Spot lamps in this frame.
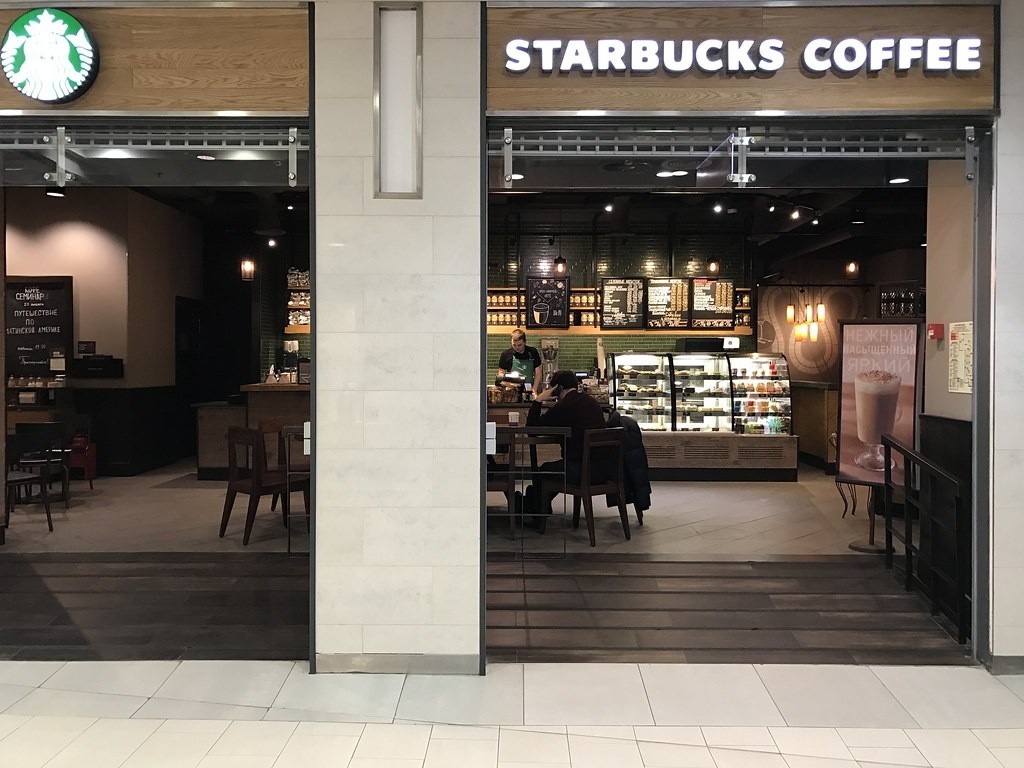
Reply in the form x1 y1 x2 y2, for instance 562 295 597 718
240 249 256 282
785 258 827 342
554 202 566 277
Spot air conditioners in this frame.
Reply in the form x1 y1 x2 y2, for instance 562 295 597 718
841 254 862 287
706 196 720 276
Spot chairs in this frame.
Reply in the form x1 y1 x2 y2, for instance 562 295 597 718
3 432 54 532
483 425 520 542
41 411 96 492
218 424 312 548
602 412 647 526
255 419 311 512
9 421 72 513
536 426 631 548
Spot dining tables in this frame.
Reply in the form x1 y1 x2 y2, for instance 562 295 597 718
487 415 572 535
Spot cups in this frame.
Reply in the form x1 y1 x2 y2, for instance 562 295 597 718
533 303 549 324
508 412 519 426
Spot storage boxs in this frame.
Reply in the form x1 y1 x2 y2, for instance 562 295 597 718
604 351 799 482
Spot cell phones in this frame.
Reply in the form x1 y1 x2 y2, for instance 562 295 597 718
551 385 563 402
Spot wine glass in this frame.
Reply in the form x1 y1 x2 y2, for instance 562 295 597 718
853 374 903 472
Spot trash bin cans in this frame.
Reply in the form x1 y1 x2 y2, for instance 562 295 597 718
68 433 96 480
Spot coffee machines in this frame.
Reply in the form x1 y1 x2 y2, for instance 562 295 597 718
540 337 559 389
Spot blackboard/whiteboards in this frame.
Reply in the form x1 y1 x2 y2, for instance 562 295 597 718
525 275 570 329
4 275 76 380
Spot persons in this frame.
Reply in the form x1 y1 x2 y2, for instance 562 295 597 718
525 369 608 535
497 328 543 401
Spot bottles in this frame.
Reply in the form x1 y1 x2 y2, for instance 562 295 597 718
36 377 43 386
8 376 16 386
18 377 26 385
27 377 36 386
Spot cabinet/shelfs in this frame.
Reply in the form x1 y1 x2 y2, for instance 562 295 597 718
282 270 312 336
485 285 758 339
